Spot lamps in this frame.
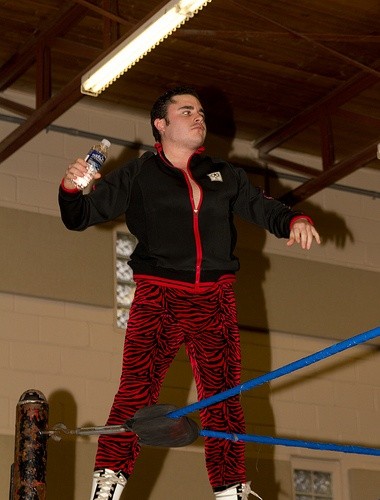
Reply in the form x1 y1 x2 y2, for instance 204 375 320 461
80 0 212 97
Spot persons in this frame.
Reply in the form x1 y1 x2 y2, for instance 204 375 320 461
56 86 321 500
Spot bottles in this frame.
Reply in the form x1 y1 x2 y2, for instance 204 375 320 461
70 139 111 189
